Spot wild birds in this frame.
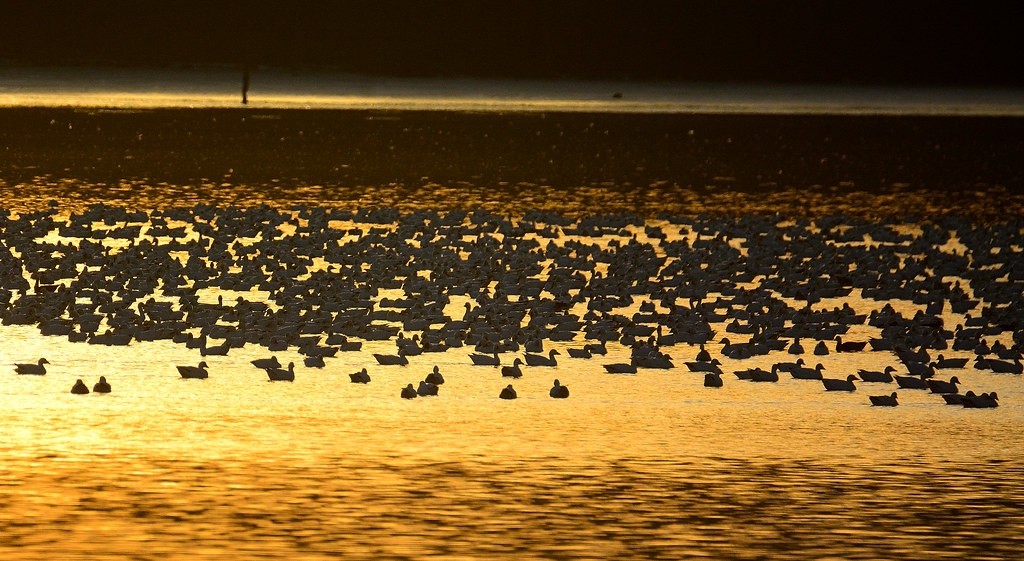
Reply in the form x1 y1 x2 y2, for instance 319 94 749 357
0 171 1024 411
